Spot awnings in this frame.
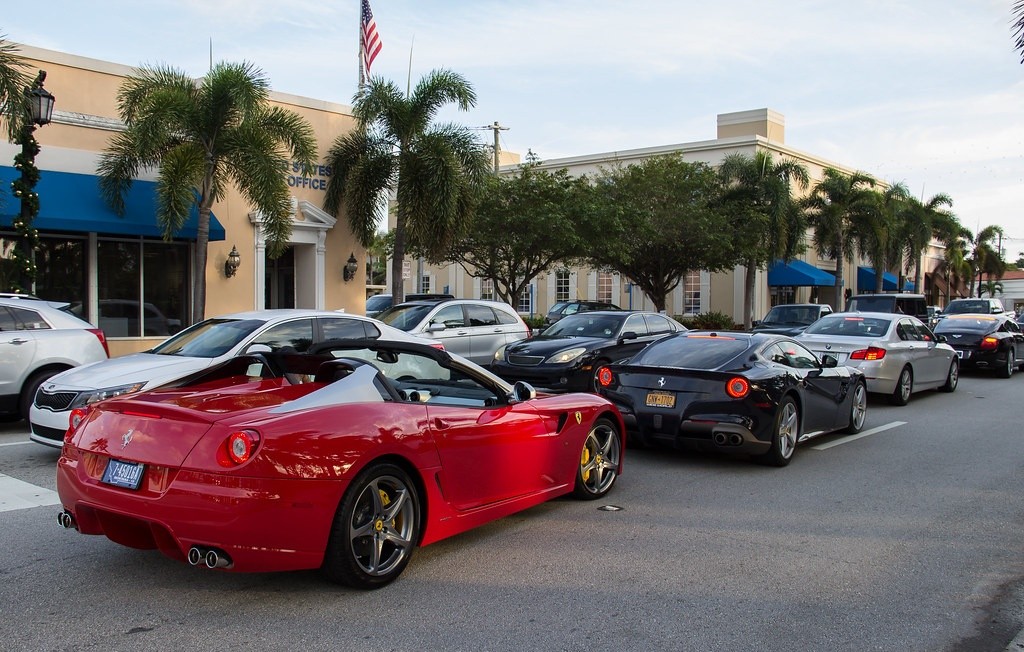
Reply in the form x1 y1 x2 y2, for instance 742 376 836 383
767 257 844 287
950 275 975 297
0 165 226 241
858 266 915 290
926 272 959 297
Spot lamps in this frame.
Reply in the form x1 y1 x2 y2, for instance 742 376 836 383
812 286 818 298
846 288 852 299
225 244 241 278
343 251 358 282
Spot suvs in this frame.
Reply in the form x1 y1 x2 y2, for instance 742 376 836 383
0 293 111 424
537 299 623 335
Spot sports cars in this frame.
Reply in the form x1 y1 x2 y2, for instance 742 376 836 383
598 330 868 468
57 341 628 593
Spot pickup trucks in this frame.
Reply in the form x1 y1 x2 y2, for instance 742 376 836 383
937 299 1016 323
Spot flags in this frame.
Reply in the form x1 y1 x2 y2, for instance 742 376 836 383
362 0 382 72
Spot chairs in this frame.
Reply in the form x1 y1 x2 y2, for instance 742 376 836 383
315 357 402 401
244 351 302 385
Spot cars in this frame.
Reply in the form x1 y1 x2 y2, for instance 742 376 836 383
489 309 688 394
63 299 170 338
367 293 455 325
926 305 942 318
751 305 834 343
791 313 962 406
29 309 447 447
933 314 1024 378
331 298 530 384
1016 313 1024 330
842 293 930 331
